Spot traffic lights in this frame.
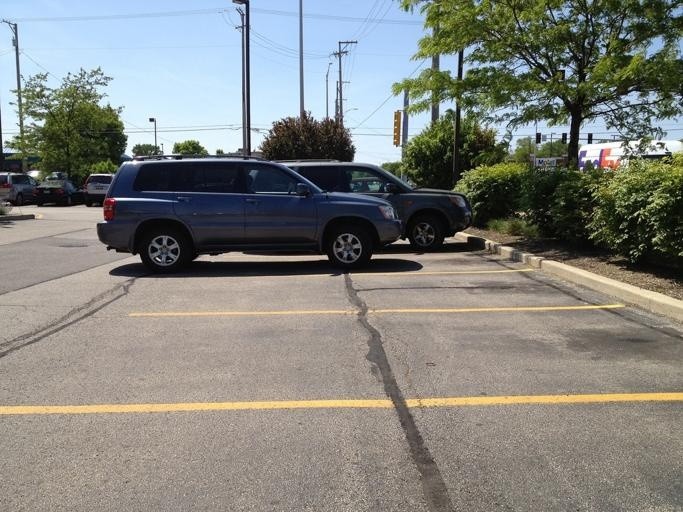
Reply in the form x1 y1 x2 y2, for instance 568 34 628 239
588 133 592 143
536 133 541 144
562 133 567 144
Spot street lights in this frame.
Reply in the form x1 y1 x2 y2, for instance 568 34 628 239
233 0 251 154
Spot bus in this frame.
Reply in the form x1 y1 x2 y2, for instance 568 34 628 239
578 140 674 173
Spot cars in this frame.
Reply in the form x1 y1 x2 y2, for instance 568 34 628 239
0 170 117 215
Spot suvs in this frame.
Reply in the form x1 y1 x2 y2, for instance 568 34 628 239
96 153 405 271
266 158 473 252
